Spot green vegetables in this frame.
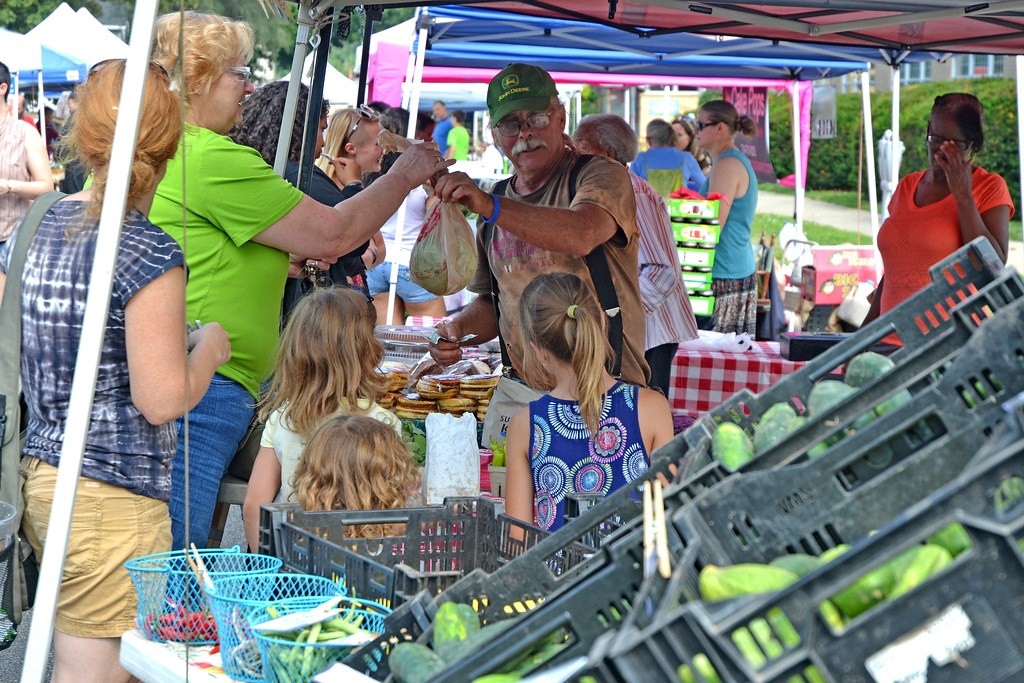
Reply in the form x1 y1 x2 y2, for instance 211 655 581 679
402 421 426 467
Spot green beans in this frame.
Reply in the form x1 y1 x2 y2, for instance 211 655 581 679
261 601 363 683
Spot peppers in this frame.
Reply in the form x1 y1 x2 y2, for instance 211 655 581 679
489 433 507 467
144 606 220 654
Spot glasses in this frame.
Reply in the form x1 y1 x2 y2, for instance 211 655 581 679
346 104 374 142
695 121 726 131
497 109 558 137
926 121 974 150
225 66 251 84
86 58 172 91
317 278 374 304
676 115 694 126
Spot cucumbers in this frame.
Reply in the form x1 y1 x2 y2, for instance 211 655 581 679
388 462 1024 683
712 352 915 471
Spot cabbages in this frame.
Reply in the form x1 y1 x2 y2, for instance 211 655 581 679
410 232 476 295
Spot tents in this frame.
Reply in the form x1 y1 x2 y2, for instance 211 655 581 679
357 16 813 228
18 0 1024 683
351 43 585 135
0 0 132 151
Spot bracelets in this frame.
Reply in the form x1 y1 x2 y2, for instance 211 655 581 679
6 179 16 194
481 193 501 223
347 179 365 189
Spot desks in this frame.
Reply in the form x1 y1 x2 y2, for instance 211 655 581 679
205 477 249 551
403 314 842 431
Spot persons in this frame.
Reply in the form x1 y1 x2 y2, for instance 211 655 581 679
628 117 707 194
367 98 470 325
500 271 680 580
696 100 758 337
855 90 1015 354
79 12 458 602
0 61 91 260
0 59 231 683
225 79 368 330
572 112 699 401
240 288 457 601
316 103 388 295
670 114 712 175
429 60 653 387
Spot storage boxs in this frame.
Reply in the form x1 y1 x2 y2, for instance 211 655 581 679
258 192 1024 683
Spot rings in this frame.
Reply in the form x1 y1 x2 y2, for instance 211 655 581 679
437 156 441 162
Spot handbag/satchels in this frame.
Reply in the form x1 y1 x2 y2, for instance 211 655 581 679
377 131 478 294
0 443 39 651
758 247 785 338
481 375 547 449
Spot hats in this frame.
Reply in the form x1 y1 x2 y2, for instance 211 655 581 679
487 63 559 128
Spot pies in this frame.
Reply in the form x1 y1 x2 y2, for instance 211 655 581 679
382 371 499 420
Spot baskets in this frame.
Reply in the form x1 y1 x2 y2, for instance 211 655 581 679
206 574 346 682
257 233 1024 683
247 596 394 683
125 547 283 643
